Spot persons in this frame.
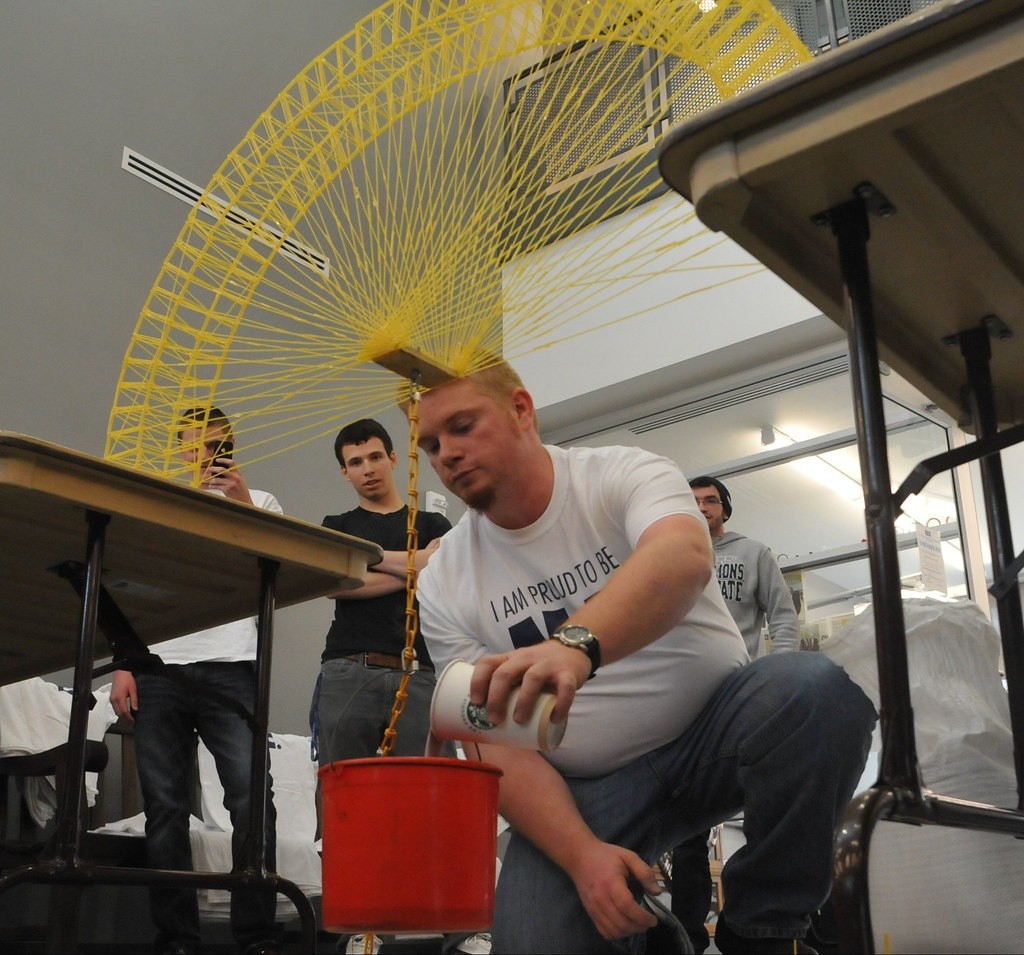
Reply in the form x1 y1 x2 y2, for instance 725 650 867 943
308 419 492 955
107 405 284 954
670 475 802 955
398 346 879 955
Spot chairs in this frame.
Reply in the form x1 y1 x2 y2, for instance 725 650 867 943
61 659 200 954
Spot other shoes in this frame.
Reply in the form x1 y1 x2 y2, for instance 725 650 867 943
247 941 281 955
160 941 194 955
714 914 819 955
642 891 696 955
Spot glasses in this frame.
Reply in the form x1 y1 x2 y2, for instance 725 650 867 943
695 496 724 505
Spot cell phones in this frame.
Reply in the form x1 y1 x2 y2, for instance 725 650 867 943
212 441 233 478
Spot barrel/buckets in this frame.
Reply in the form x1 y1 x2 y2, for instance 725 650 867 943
313 668 506 934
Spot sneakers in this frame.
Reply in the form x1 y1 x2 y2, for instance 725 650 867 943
455 933 492 955
346 934 383 955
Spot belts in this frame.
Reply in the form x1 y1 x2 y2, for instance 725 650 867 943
345 652 434 671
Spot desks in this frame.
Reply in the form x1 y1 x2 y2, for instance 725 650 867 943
651 2 1024 955
1 429 386 955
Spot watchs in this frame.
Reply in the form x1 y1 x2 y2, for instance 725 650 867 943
549 625 601 680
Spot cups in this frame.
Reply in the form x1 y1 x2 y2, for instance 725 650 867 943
430 658 566 752
